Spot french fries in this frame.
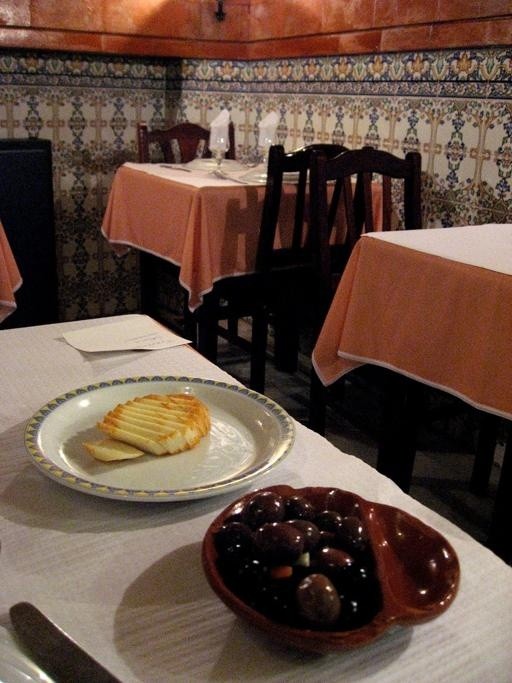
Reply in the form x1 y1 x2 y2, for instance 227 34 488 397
83 390 213 464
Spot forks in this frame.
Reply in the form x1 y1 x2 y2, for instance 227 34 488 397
214 170 247 185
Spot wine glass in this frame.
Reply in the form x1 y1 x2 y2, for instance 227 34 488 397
262 131 281 176
208 132 230 174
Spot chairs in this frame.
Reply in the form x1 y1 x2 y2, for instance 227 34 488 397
308 150 422 438
219 143 376 395
136 121 236 314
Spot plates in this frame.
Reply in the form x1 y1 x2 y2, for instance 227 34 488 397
23 374 297 503
199 483 462 656
284 171 309 181
194 157 233 171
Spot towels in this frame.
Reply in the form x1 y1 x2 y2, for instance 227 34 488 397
208 107 231 152
258 110 280 150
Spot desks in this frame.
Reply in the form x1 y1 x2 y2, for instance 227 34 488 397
1 140 58 325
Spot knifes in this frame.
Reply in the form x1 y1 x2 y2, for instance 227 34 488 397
159 163 192 172
7 600 122 683
